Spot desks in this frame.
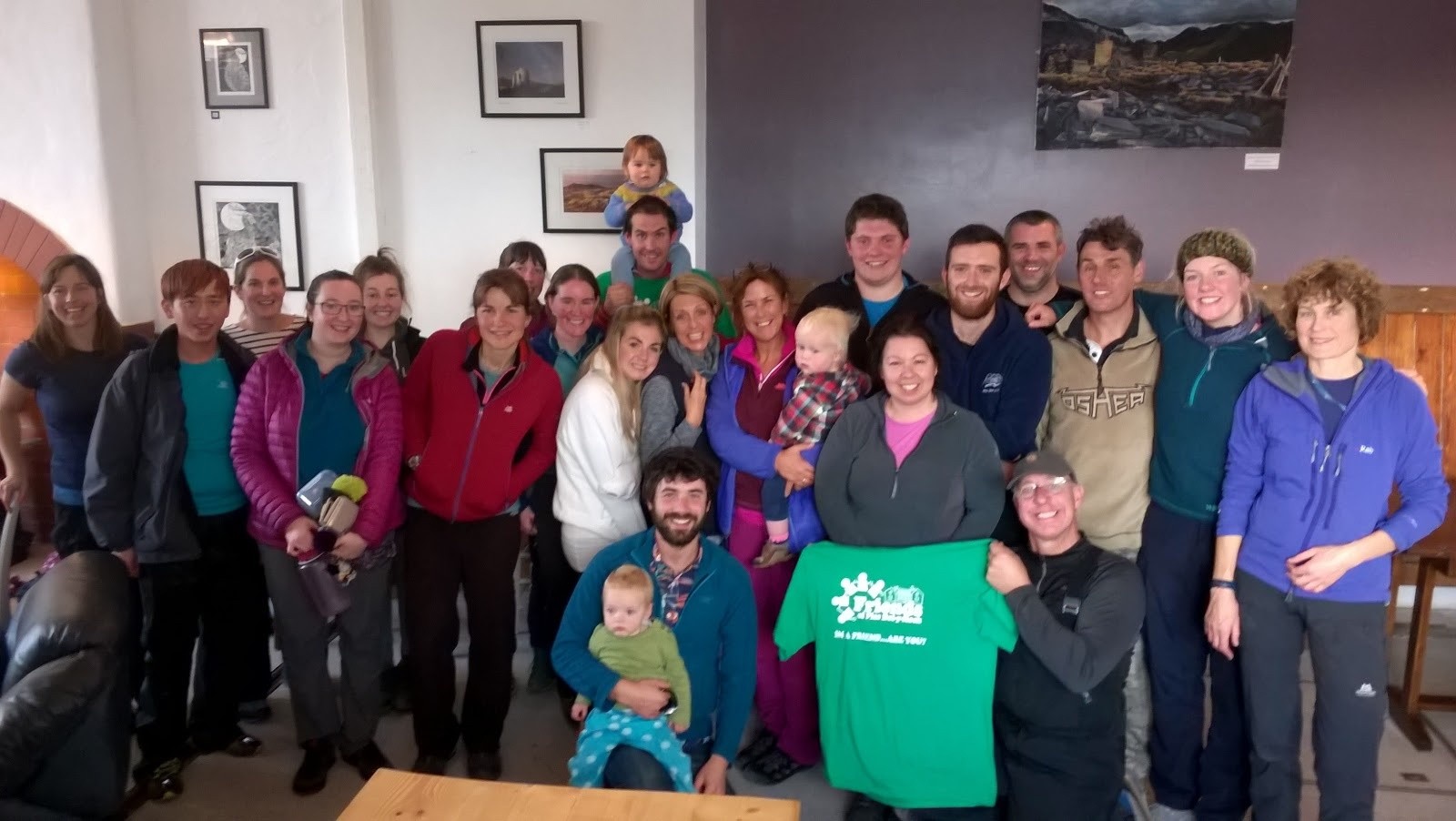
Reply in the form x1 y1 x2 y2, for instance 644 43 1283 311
335 768 801 821
1388 478 1456 753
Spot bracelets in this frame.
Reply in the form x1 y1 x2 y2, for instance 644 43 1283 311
1209 579 1238 590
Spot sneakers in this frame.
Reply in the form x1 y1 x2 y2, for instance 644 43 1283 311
139 749 184 803
192 723 262 757
526 647 557 692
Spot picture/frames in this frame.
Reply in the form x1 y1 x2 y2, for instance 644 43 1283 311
475 19 585 118
195 181 306 291
540 148 635 233
199 28 269 109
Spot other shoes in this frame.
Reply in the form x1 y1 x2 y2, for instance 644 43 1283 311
410 716 460 774
736 733 816 784
461 710 503 780
292 738 336 796
337 723 387 782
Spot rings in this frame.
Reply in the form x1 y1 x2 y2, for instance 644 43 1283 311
803 478 807 484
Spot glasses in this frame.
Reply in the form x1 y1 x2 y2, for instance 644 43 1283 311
234 246 281 271
316 300 366 316
1019 477 1066 497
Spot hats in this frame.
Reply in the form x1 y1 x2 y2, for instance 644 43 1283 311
1006 451 1074 491
1176 227 1256 282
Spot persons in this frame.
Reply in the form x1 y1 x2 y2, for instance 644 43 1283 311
605 135 695 292
0 195 1449 821
